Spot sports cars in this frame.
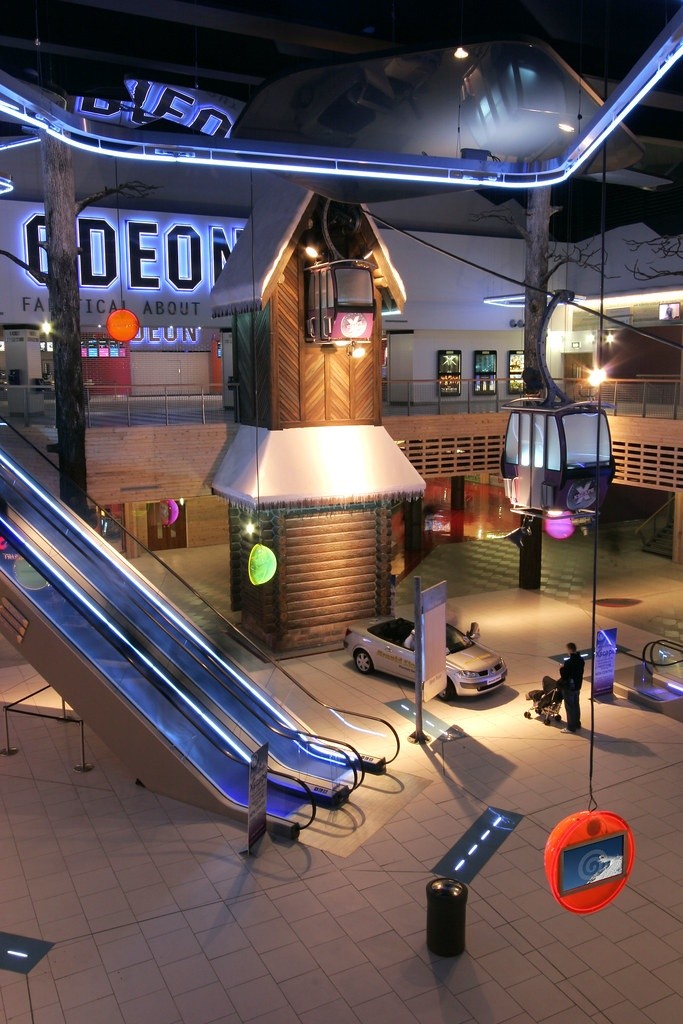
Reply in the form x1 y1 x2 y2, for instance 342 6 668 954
343 614 508 701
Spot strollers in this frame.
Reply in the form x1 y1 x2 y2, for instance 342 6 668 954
524 674 565 725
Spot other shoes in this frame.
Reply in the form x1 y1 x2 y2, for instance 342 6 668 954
561 728 573 734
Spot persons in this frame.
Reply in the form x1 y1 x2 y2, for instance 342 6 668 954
557 642 585 733
